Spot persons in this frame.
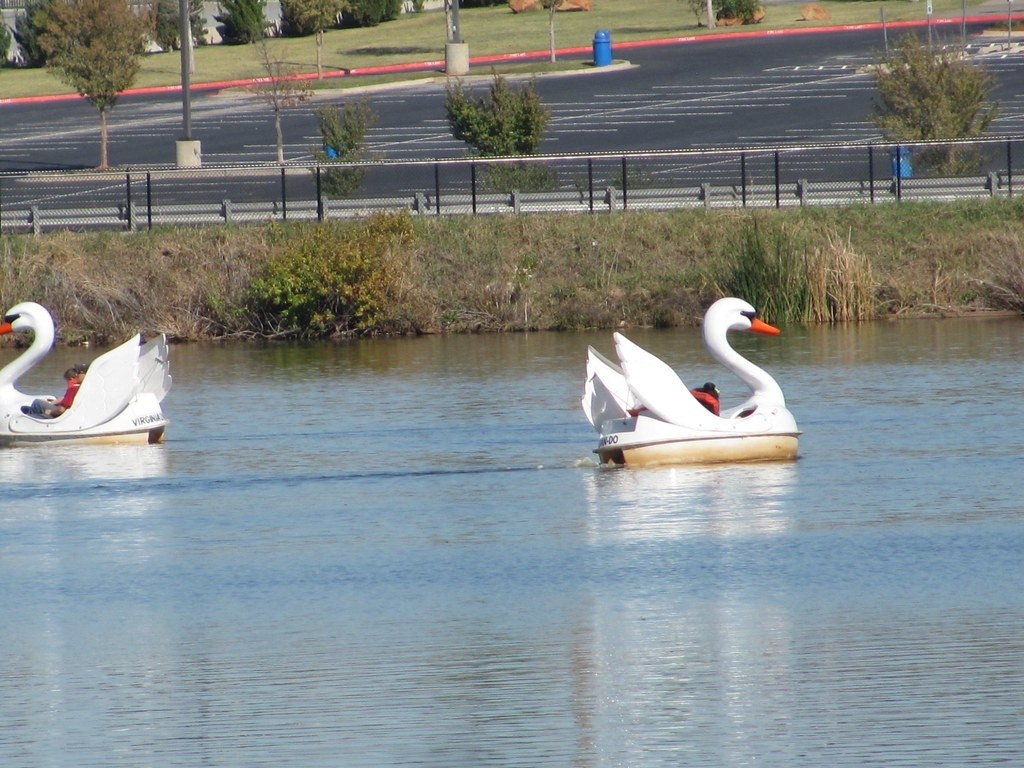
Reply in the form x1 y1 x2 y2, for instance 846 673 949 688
22 364 90 418
41 368 77 404
690 382 720 417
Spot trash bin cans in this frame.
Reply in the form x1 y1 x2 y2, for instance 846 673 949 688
890 147 913 179
593 31 611 67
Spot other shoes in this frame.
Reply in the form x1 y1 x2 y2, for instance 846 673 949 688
21 405 30 412
